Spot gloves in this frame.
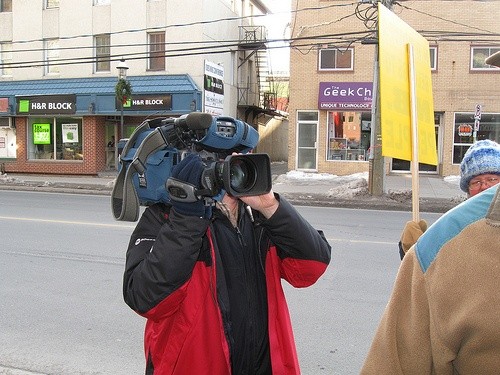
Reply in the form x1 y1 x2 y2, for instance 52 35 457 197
164 153 205 217
400 218 428 254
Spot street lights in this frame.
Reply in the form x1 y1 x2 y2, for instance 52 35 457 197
116 58 129 139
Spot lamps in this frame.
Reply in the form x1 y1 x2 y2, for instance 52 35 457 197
190 100 196 111
88 103 93 113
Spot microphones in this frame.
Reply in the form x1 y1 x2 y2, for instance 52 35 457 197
174 113 212 130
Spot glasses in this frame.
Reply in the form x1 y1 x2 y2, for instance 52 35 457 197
468 177 500 191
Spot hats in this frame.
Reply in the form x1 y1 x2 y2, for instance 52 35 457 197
460 139 500 192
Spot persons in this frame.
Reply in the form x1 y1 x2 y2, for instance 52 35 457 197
122 134 332 375
397 138 500 262
360 181 500 375
